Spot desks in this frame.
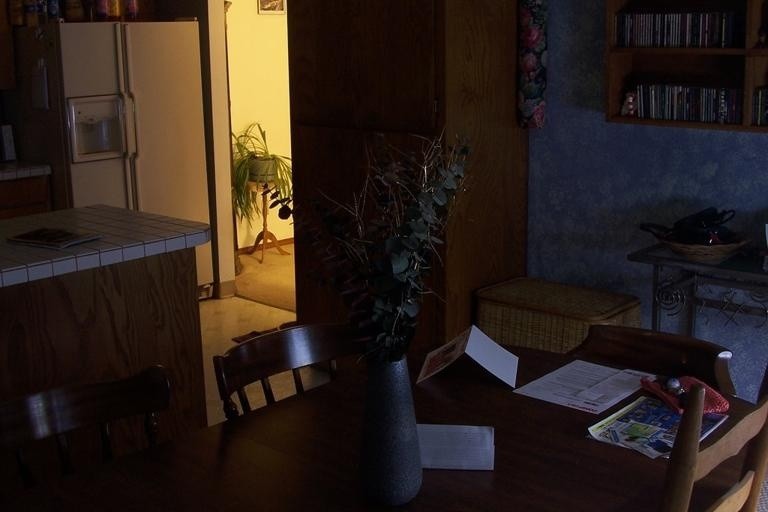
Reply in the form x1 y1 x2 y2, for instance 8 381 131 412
626 239 768 338
3 339 755 507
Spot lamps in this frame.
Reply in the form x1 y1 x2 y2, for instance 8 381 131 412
515 0 547 130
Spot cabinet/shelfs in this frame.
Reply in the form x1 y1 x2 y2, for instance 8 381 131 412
605 0 768 133
0 0 17 89
286 0 528 354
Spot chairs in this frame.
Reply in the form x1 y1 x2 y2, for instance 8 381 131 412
210 323 348 423
563 324 737 397
2 363 172 489
658 382 768 512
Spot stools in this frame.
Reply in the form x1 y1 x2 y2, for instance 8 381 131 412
247 180 289 263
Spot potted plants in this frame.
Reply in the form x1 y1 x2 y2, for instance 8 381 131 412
261 129 469 502
231 122 293 230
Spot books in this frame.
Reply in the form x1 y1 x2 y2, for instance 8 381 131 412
6 229 103 250
416 324 519 389
614 10 768 126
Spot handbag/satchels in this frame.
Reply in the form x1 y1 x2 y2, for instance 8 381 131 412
641 376 730 417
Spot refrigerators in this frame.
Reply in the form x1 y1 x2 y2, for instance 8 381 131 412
15 17 214 298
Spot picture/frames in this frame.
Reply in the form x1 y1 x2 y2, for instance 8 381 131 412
258 0 286 15
67 94 125 164
